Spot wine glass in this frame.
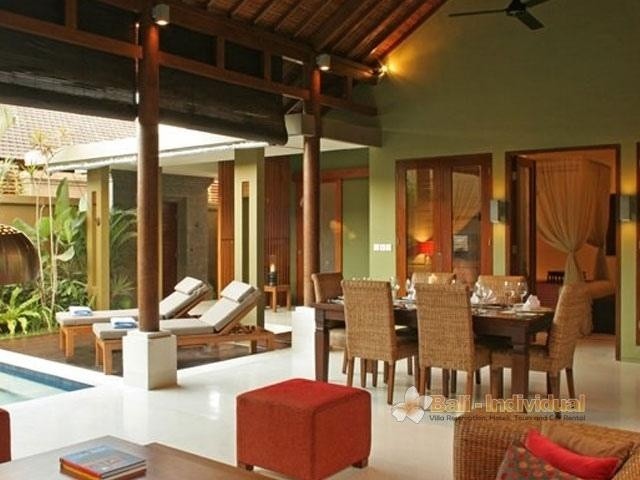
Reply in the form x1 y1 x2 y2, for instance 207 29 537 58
389 275 529 314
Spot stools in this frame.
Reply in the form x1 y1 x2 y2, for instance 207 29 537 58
237 378 371 479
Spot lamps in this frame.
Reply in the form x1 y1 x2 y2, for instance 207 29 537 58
416 240 435 267
152 4 170 26
315 54 332 72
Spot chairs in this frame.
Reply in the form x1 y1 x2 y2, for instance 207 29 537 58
311 272 583 419
55 275 274 376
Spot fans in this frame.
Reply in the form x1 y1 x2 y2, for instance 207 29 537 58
448 1 550 31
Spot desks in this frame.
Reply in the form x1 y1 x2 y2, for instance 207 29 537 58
1 435 235 479
264 284 292 312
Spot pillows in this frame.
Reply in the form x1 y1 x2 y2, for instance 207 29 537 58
495 422 633 480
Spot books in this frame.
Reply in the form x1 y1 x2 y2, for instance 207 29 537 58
60 461 147 480
59 445 147 479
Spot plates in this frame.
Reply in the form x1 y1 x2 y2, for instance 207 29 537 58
515 306 553 313
